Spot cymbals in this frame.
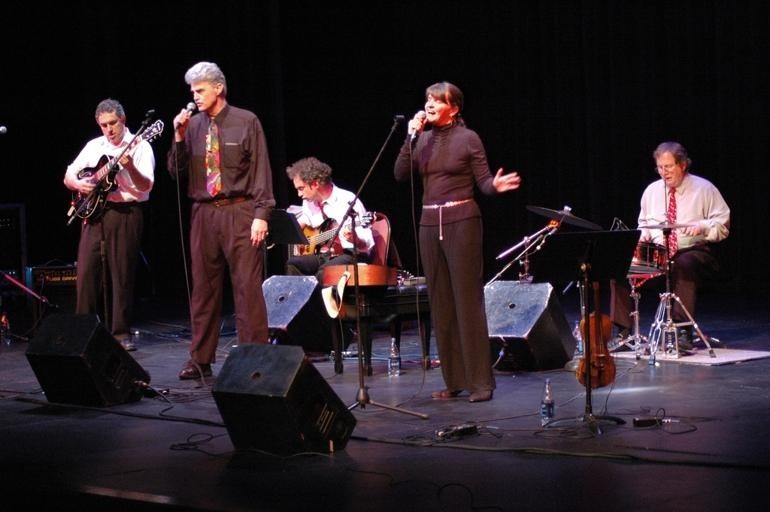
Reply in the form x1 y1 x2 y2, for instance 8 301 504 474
529 205 602 231
639 223 697 228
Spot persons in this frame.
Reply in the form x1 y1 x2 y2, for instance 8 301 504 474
636 141 730 351
169 61 275 379
394 80 520 403
64 98 155 351
284 156 376 363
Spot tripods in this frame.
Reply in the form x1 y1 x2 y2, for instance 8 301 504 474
326 114 429 420
608 233 716 365
543 274 627 436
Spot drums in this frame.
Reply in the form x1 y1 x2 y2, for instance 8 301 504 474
630 240 670 273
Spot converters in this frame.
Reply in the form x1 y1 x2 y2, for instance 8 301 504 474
434 424 478 436
633 417 663 427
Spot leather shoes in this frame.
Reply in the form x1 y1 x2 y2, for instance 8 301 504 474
677 330 695 351
430 388 463 399
179 361 213 380
468 392 494 403
120 339 138 352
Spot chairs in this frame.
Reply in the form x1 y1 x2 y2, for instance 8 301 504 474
326 213 431 376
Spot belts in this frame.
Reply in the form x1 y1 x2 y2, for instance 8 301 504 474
205 194 250 208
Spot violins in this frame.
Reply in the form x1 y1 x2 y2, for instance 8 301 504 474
577 280 616 390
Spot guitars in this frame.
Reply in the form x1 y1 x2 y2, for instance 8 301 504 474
292 212 377 258
71 119 165 221
322 264 414 287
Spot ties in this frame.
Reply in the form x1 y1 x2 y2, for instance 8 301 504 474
204 116 222 198
318 201 343 257
663 187 678 261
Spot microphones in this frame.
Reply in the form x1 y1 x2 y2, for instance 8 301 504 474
175 102 196 130
410 111 426 144
145 109 156 117
536 228 559 251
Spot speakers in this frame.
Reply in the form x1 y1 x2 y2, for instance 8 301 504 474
483 281 577 372
262 275 355 355
212 345 358 450
25 314 151 408
